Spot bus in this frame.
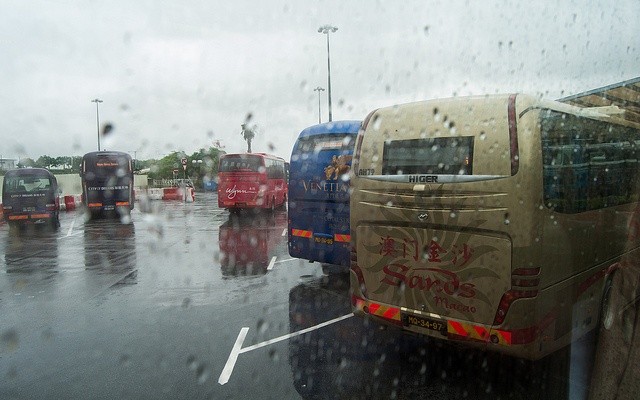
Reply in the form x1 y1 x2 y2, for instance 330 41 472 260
2 168 62 228
218 152 289 215
79 149 135 210
350 93 640 361
288 120 363 267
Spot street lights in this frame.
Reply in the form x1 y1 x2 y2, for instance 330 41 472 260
318 24 339 121
91 99 103 151
314 86 325 124
192 160 203 172
